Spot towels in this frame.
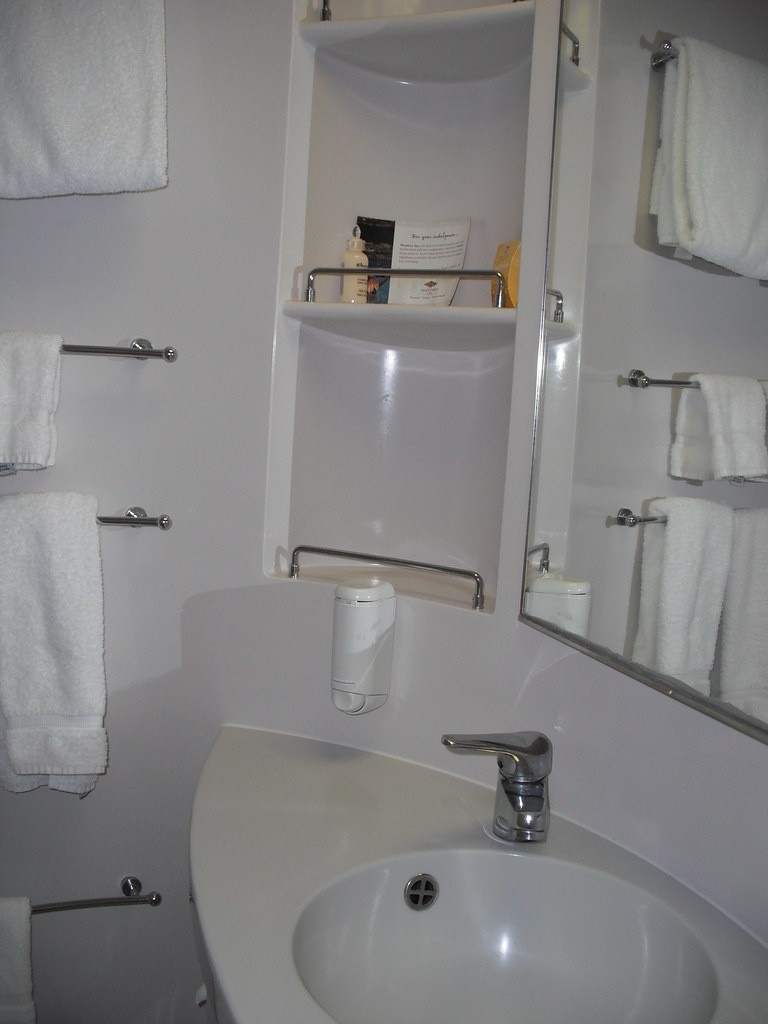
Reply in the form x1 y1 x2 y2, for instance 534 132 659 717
624 494 735 699
667 373 768 483
648 35 768 281
0 896 37 1024
719 506 768 728
2 492 108 796
0 330 62 476
0 0 168 200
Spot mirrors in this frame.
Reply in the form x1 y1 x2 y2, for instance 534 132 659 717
515 0 767 744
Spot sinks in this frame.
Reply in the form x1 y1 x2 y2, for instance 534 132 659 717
290 849 720 1024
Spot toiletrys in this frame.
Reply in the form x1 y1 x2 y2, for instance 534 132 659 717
338 225 370 303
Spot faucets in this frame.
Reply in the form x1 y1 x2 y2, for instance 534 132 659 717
440 730 553 844
